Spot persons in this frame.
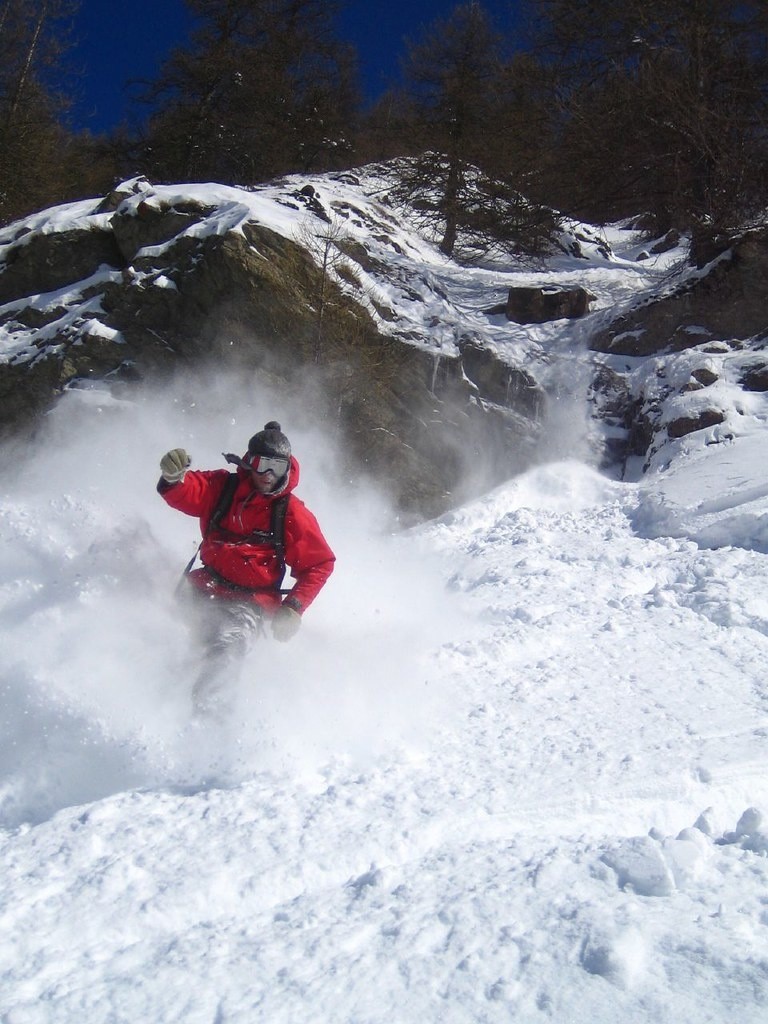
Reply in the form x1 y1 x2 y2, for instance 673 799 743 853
156 422 336 720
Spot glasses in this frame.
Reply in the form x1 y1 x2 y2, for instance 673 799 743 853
246 453 290 479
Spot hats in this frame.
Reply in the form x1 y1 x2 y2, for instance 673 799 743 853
248 421 291 461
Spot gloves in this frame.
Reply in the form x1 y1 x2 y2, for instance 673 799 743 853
271 606 302 644
160 448 192 486
